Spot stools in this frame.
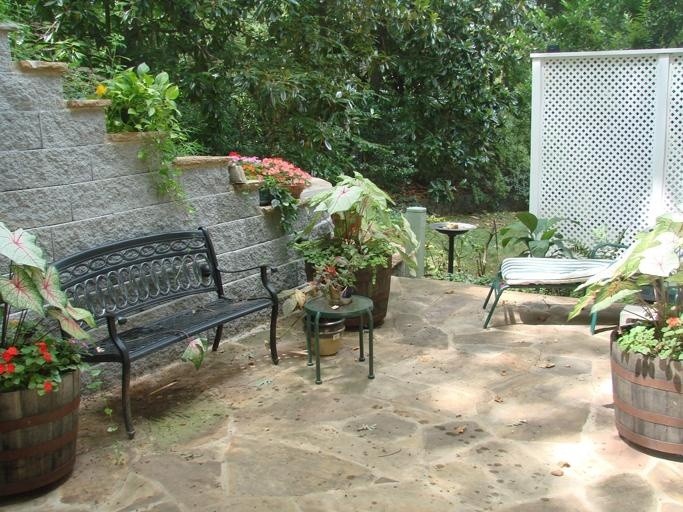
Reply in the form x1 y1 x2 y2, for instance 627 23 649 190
302 296 376 384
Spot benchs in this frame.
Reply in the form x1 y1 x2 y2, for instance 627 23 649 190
43 226 283 440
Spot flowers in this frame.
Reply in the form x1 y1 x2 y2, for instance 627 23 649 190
568 210 683 360
0 220 121 436
228 153 311 185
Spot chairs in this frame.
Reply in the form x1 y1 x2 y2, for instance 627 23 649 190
483 242 628 336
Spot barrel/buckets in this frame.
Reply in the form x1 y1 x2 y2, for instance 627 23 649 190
303 315 346 358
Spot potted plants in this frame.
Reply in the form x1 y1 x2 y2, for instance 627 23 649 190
287 175 419 356
258 183 305 206
88 62 197 215
